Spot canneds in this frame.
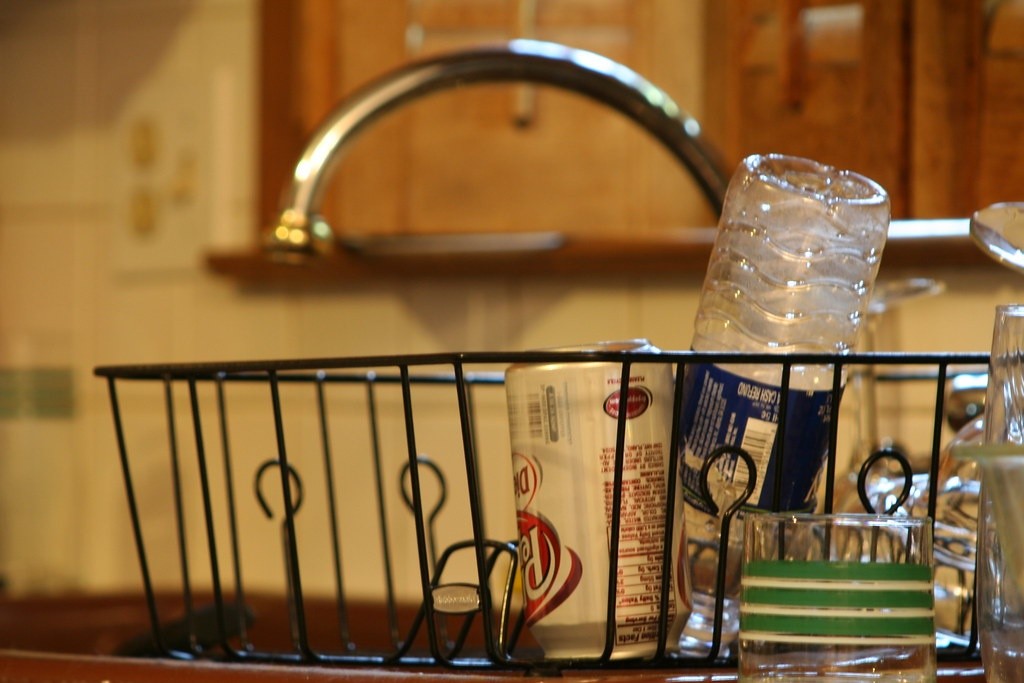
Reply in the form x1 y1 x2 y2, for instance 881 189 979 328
503 340 694 659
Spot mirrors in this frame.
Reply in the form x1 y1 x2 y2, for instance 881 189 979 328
210 0 1024 278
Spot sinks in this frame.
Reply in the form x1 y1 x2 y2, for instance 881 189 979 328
0 597 551 682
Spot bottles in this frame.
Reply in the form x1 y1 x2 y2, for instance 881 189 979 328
677 150 893 664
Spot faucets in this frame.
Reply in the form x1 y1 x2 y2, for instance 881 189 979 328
248 31 742 270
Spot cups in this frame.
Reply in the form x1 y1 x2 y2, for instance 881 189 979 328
736 501 941 683
978 301 1024 683
